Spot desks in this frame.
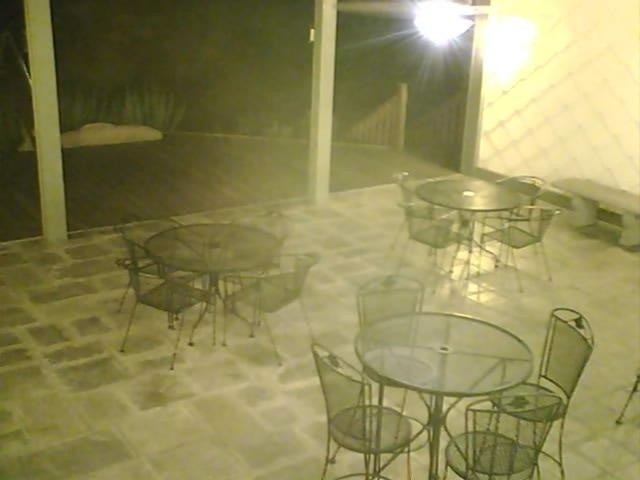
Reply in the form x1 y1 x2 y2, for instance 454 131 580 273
143 225 284 348
354 310 534 478
416 175 530 277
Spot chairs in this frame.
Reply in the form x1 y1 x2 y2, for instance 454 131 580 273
398 172 452 257
479 202 559 293
352 272 425 411
399 199 467 275
222 247 320 369
117 255 214 372
115 213 190 330
446 390 564 480
477 303 594 468
309 341 415 478
485 173 547 268
216 205 294 327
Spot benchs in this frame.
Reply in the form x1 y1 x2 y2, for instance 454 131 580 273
554 180 640 246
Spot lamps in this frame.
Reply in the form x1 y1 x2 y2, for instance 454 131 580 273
411 0 473 45
